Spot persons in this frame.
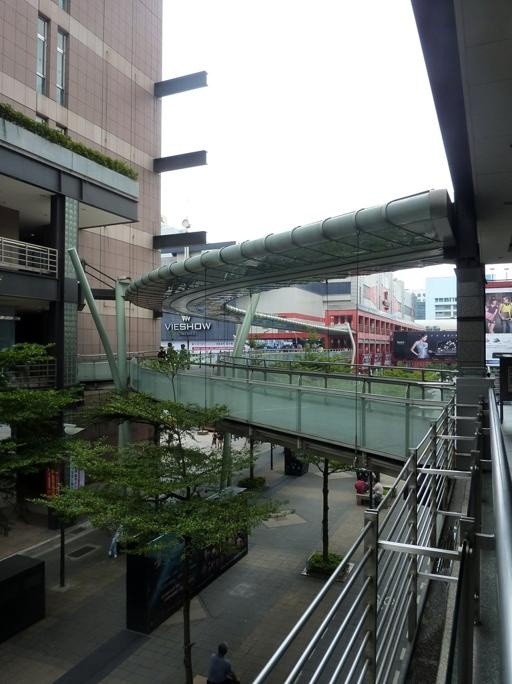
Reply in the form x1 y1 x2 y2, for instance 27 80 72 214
274 340 324 352
216 431 223 449
205 642 236 683
107 522 123 559
166 342 177 370
497 295 512 333
371 477 383 509
353 475 370 494
178 343 187 370
411 333 433 359
210 431 217 448
486 294 499 333
157 346 166 366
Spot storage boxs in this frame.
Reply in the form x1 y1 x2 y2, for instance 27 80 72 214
355 484 398 509
